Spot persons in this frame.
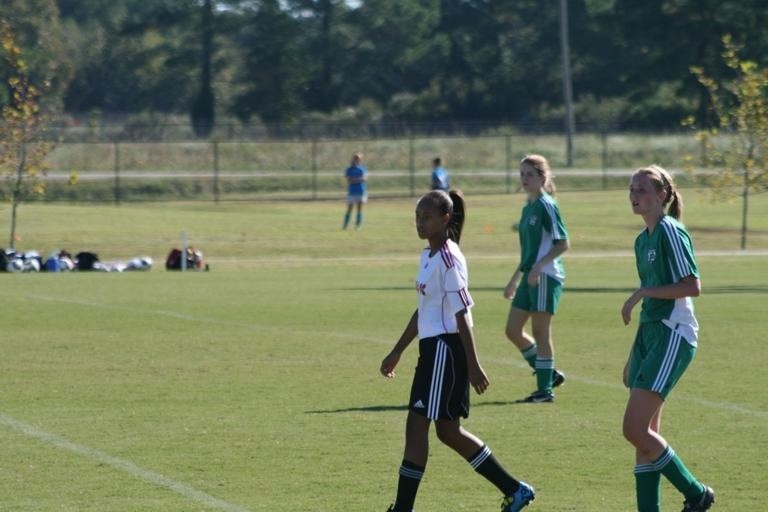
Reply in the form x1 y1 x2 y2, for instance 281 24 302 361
429 157 449 192
619 163 718 512
379 186 539 512
341 153 368 230
503 151 571 404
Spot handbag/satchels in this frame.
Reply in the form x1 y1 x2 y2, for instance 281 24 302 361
1 248 153 272
167 247 201 269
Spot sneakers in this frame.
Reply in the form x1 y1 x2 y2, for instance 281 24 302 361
515 371 564 404
501 482 534 512
680 482 716 512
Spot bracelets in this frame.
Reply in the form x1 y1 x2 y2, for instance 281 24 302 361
532 266 538 273
